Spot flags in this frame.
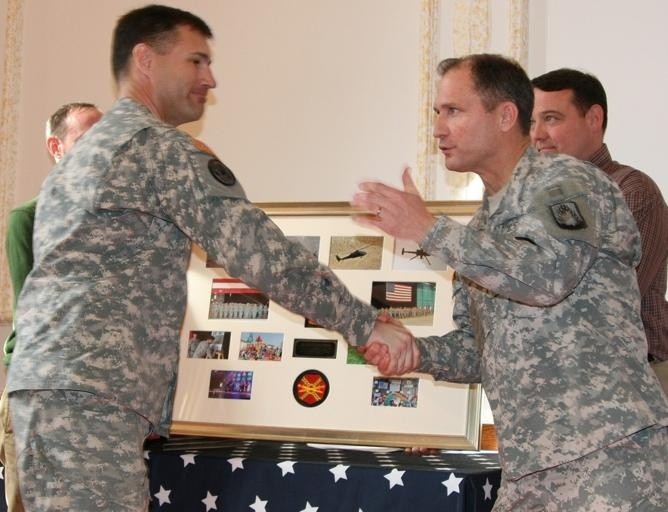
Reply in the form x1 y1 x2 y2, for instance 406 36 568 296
385 282 413 302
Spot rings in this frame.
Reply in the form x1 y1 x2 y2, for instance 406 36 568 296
375 205 383 221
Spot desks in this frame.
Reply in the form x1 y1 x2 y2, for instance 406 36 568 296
144 436 502 512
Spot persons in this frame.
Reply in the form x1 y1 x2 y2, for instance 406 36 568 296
193 336 215 360
530 69 666 397
374 306 431 318
208 301 268 319
188 333 199 358
348 54 668 512
230 378 237 391
373 389 381 406
6 6 419 512
384 390 393 406
1 103 103 512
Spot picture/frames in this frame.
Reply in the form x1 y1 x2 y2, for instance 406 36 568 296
167 201 487 451
